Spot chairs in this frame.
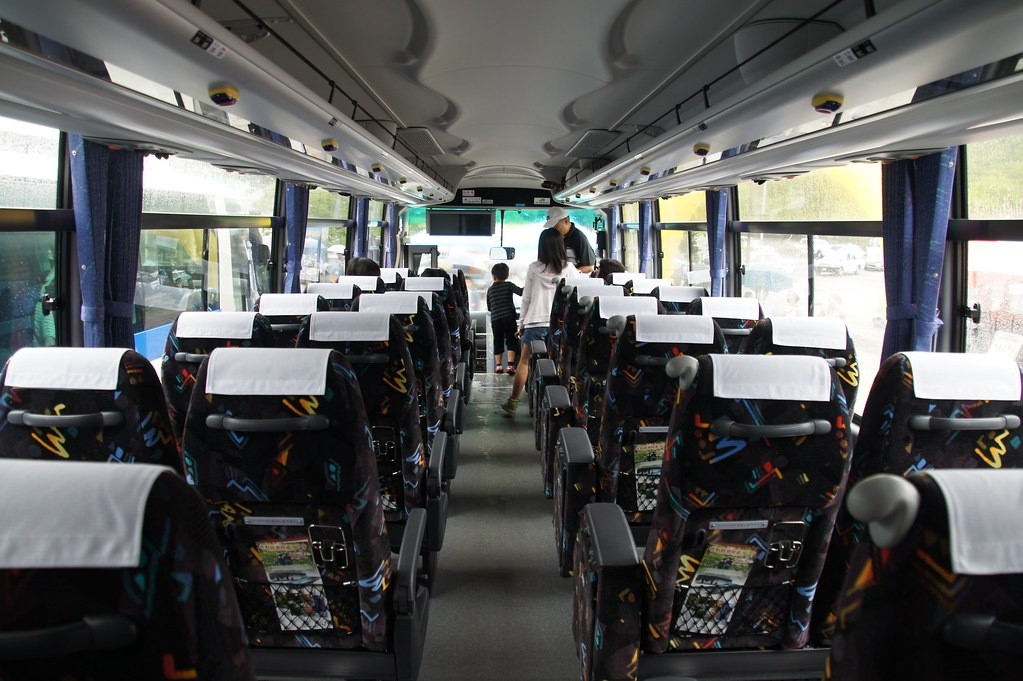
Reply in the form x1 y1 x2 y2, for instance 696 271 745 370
0 259 1023 681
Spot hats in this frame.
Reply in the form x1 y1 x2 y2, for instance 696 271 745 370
543 207 569 228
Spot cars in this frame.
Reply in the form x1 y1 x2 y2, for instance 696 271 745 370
319 245 382 282
441 251 491 288
799 237 885 276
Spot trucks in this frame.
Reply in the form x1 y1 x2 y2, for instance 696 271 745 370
298 226 330 282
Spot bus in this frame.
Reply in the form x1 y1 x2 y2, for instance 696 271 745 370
0 173 270 376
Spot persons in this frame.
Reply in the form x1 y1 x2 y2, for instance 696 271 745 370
500 207 625 415
487 263 524 375
33 266 56 347
346 257 451 278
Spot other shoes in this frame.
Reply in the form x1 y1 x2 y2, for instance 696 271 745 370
502 398 519 414
496 365 503 373
506 365 516 374
